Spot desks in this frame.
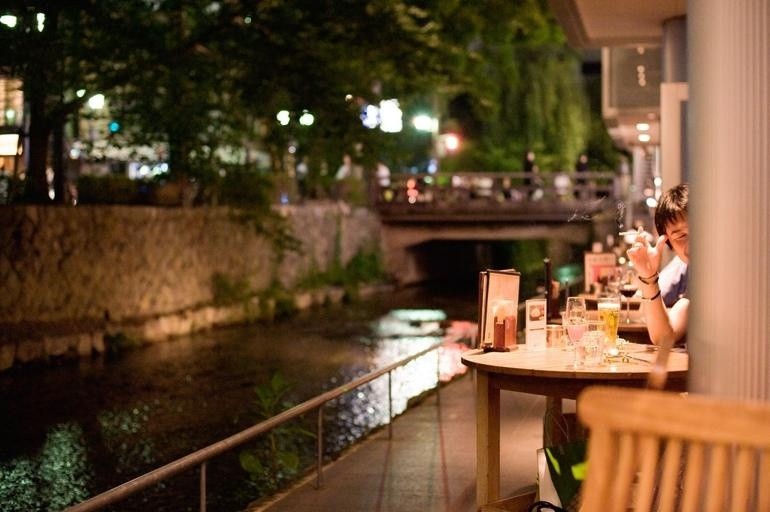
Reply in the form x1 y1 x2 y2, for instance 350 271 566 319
463 290 691 501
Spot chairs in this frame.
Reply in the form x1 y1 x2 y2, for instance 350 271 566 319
574 386 769 512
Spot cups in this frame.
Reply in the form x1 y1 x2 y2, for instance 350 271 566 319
546 325 566 349
596 297 621 356
576 320 604 369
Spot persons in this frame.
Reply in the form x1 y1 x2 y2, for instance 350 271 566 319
525 150 543 202
333 156 352 180
377 161 391 201
575 154 588 197
626 181 690 349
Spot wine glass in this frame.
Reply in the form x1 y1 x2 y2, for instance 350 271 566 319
619 269 638 324
563 296 588 352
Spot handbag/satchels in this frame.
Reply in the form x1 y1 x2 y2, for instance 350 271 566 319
537 441 586 512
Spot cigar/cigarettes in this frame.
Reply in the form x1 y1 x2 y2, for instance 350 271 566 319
618 232 641 236
